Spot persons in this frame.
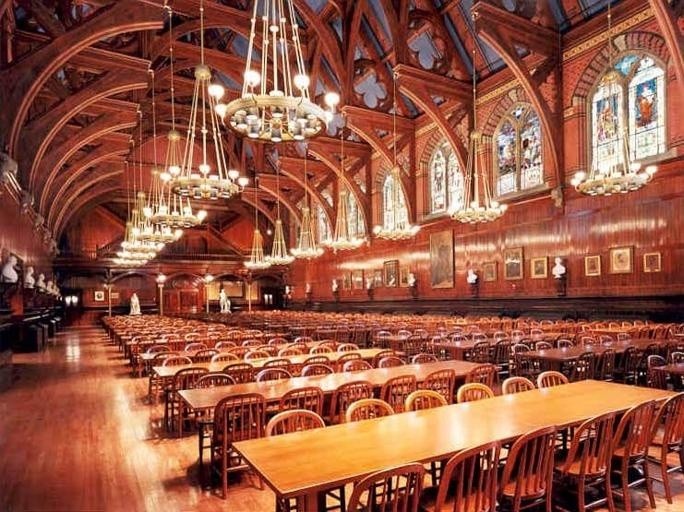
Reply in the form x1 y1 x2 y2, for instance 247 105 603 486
131 292 140 314
332 280 337 293
0 255 63 299
409 273 415 287
552 256 565 278
467 269 477 284
641 83 654 118
219 287 228 313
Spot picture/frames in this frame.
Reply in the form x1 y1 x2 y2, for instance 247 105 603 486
481 261 498 282
584 255 602 277
373 269 383 289
503 247 525 281
609 245 634 275
399 265 411 288
430 230 455 289
530 256 549 280
351 269 364 291
642 252 662 273
95 291 104 302
383 260 400 288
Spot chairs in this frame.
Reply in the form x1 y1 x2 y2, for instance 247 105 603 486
102 307 684 512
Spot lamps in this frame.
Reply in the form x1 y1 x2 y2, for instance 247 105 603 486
242 174 274 269
114 1 209 268
371 71 420 240
288 143 324 261
157 1 250 204
449 49 509 225
208 0 344 143
327 129 370 252
266 148 296 267
